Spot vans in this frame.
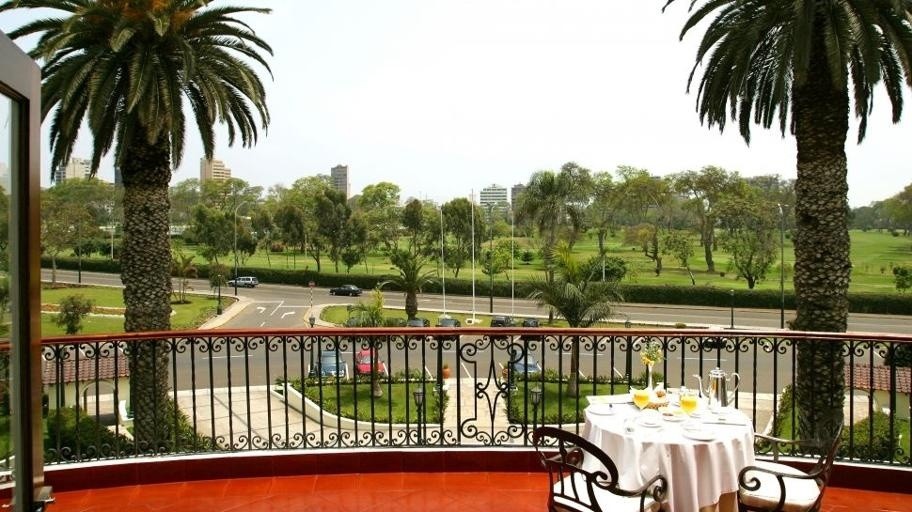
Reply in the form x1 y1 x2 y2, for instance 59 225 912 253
229 276 260 289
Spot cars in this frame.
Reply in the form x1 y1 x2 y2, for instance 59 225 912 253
405 317 430 327
354 348 385 377
436 318 461 341
522 318 544 341
315 348 346 378
510 350 540 374
330 284 363 296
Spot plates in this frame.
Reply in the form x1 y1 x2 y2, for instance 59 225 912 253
634 416 687 427
587 404 615 416
684 431 718 442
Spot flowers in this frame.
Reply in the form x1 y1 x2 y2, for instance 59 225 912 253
639 339 666 366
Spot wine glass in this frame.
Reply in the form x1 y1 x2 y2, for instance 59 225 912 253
680 389 700 427
632 390 650 413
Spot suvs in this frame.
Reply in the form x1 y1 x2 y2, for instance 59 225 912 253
490 316 516 339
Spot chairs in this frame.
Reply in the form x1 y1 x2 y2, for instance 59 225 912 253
533 426 668 511
738 423 844 511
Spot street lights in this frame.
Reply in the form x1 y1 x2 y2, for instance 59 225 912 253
233 201 252 296
479 200 510 312
775 203 788 328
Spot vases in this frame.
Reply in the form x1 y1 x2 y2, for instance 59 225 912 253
647 363 654 390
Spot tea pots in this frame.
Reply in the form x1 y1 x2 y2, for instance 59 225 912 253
693 368 741 411
